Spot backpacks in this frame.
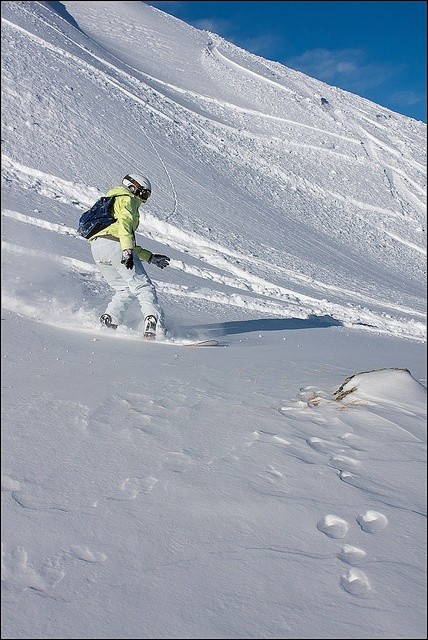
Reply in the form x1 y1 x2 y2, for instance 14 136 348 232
77 194 131 238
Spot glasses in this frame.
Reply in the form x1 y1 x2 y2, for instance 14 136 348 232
125 176 148 199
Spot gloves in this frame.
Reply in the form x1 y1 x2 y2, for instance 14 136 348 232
149 254 170 269
121 249 134 270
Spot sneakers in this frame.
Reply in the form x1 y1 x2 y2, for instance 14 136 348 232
144 315 169 340
100 315 117 330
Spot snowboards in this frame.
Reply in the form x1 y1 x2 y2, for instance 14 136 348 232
133 340 218 346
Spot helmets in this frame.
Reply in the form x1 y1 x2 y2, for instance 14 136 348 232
123 174 151 195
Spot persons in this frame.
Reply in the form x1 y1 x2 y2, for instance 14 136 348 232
88 173 171 340
